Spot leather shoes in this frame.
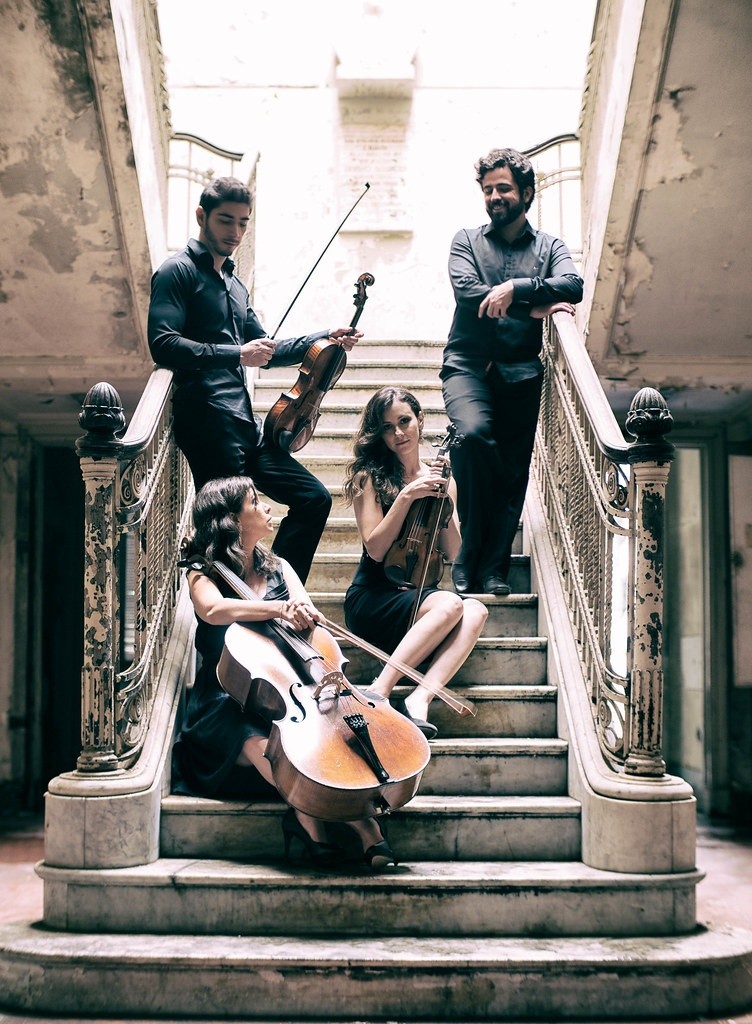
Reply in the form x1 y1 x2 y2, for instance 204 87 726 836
451 564 472 593
472 575 510 594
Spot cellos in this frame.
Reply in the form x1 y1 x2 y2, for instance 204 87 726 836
194 539 426 814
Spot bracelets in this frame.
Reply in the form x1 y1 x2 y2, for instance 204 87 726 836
281 600 286 623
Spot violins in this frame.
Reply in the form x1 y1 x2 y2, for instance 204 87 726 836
380 423 464 586
267 273 375 447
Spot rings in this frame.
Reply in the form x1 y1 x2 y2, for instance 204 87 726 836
304 613 312 621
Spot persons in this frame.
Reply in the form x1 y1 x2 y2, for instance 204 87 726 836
343 387 489 741
187 477 397 870
147 177 364 587
438 148 585 594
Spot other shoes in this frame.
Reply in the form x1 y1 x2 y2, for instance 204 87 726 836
394 700 438 740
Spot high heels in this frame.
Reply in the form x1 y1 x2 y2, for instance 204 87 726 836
363 840 398 871
281 807 346 870
